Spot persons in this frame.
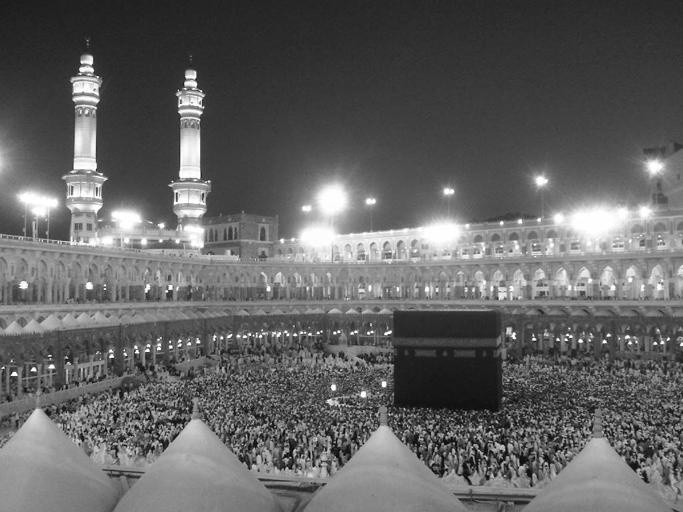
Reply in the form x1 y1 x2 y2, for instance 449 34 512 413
0 289 681 488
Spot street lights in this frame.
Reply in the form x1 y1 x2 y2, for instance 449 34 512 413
314 185 347 229
18 188 59 242
442 187 455 221
365 198 376 233
109 206 140 251
646 159 664 213
301 205 311 230
182 223 205 255
535 175 549 219
